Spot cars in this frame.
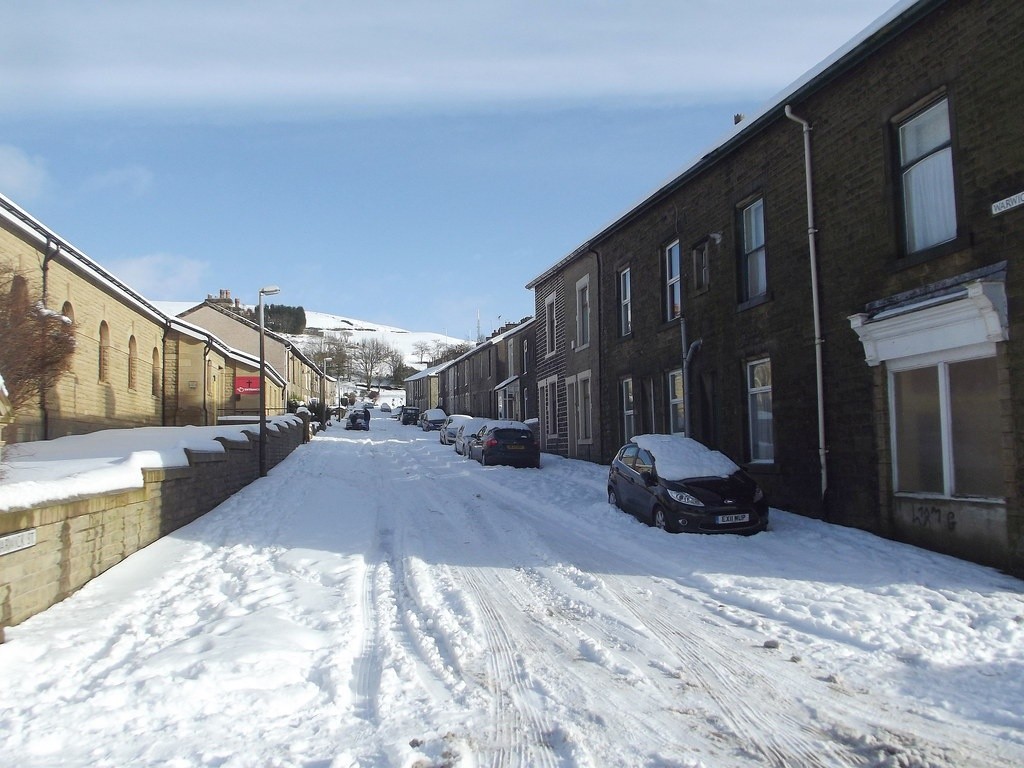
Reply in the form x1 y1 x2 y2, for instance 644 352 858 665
344 409 367 431
364 403 374 409
390 404 448 432
607 443 769 537
455 417 493 456
380 403 391 412
439 414 473 445
469 420 541 469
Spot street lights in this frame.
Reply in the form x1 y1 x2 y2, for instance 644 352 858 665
338 376 343 422
258 285 281 476
322 357 333 432
